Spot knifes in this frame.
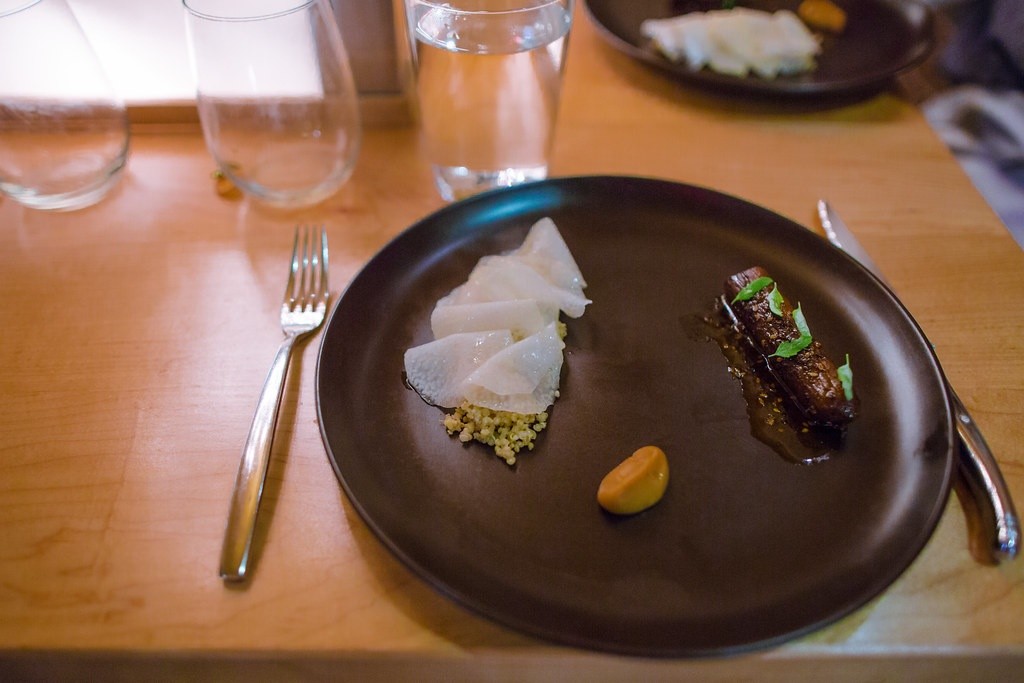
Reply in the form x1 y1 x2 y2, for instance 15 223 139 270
818 198 1022 562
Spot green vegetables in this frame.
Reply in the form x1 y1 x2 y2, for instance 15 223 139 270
732 277 854 402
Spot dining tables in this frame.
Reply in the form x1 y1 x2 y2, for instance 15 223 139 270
0 2 1024 683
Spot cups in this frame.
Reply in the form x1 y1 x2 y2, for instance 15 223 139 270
0 0 130 214
404 0 573 201
180 0 363 210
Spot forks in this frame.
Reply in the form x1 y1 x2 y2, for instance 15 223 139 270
218 226 331 581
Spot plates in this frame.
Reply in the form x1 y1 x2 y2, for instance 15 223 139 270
312 175 959 661
588 0 947 101
